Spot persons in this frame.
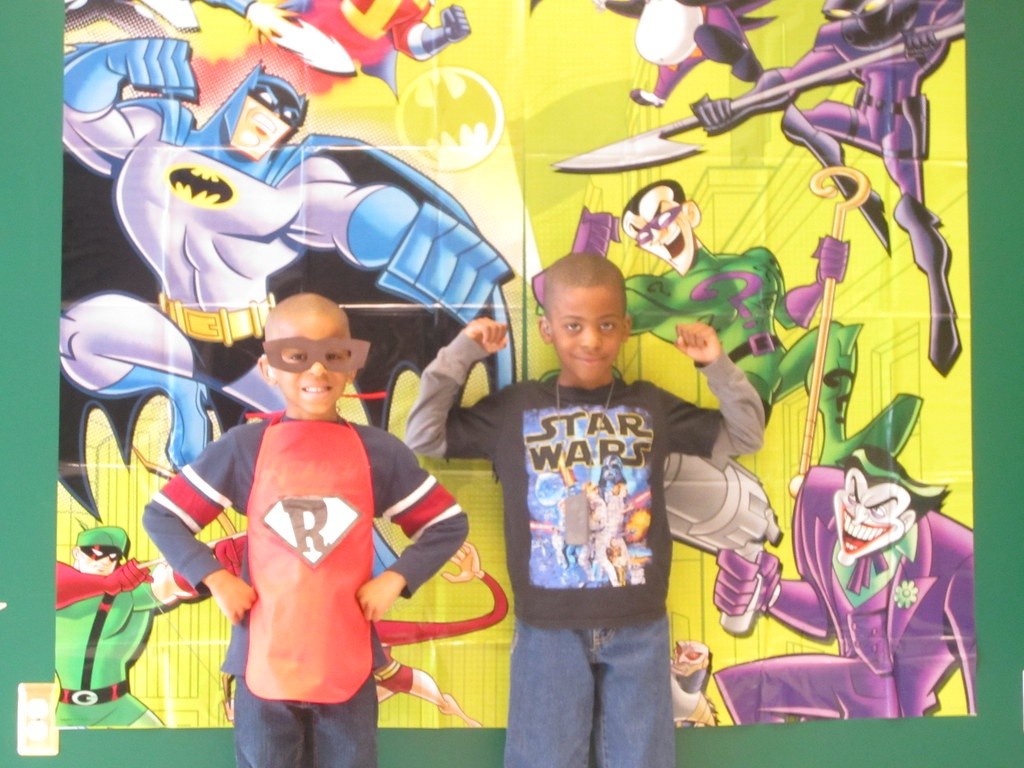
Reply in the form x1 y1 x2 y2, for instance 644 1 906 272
144 294 470 768
406 253 764 768
